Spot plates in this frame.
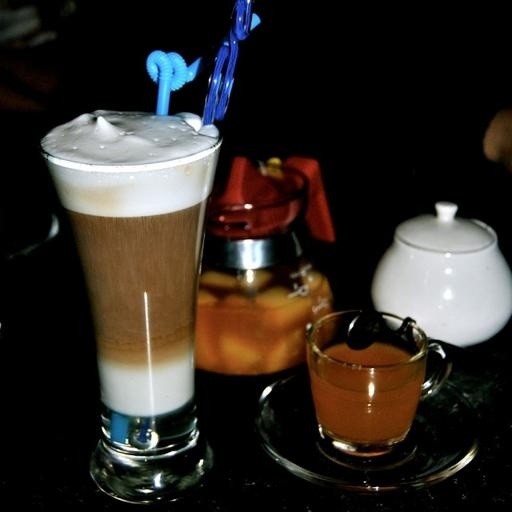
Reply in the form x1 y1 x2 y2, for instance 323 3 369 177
253 367 493 490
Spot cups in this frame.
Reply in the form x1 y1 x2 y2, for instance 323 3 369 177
43 112 226 503
303 312 450 461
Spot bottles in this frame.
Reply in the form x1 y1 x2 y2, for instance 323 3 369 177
371 201 512 346
193 157 339 376
